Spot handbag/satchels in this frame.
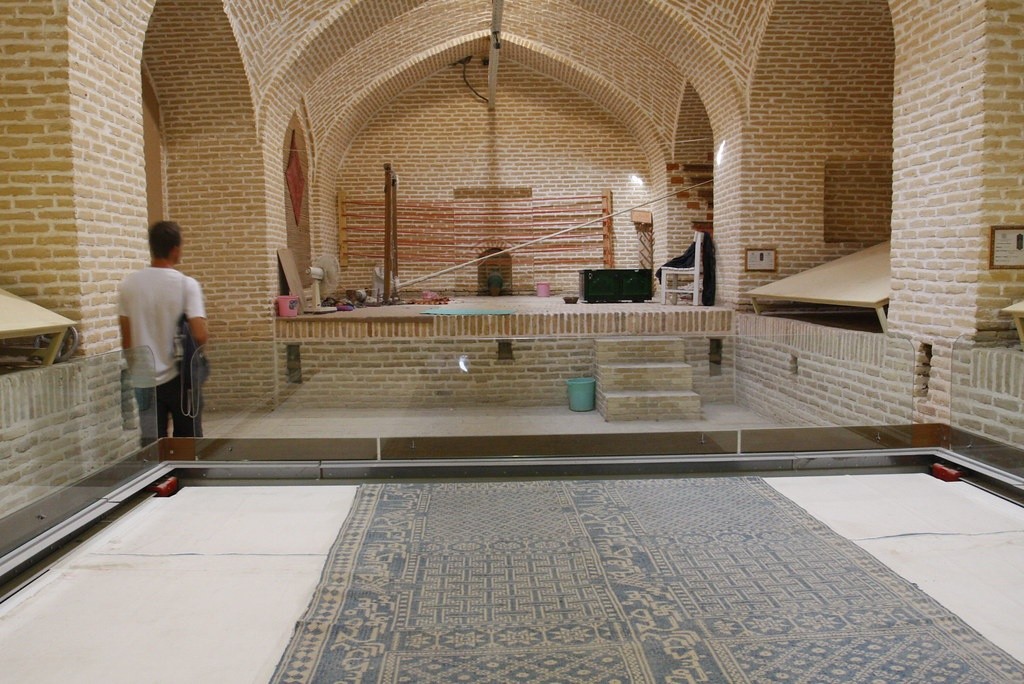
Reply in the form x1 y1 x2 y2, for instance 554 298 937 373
178 319 208 382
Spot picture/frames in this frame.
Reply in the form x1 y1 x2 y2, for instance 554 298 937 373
745 248 776 272
989 225 1024 269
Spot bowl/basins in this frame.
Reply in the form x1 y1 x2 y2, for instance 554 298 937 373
563 296 579 304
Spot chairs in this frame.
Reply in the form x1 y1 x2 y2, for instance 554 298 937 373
655 231 716 306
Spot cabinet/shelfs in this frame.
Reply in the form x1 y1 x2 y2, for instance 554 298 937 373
578 269 652 302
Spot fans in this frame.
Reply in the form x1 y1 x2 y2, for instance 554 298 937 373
303 254 340 312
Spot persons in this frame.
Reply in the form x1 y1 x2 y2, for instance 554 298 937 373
115 220 207 450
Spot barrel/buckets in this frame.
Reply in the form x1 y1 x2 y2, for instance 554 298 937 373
277 296 299 317
537 283 549 297
567 378 595 412
